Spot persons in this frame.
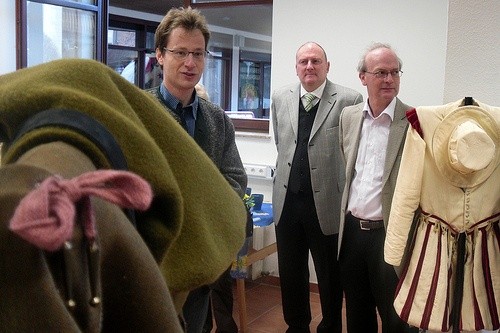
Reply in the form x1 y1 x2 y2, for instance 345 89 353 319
340 42 420 333
270 40 363 333
142 6 247 333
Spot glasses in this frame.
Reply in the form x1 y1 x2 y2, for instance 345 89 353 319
363 70 404 79
163 47 210 61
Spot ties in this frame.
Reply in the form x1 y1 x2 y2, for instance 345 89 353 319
303 93 317 111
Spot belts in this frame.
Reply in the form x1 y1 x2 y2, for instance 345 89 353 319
347 216 384 230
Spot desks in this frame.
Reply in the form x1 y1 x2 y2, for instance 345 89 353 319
232 241 278 333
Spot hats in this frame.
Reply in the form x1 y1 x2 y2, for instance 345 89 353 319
432 106 500 187
